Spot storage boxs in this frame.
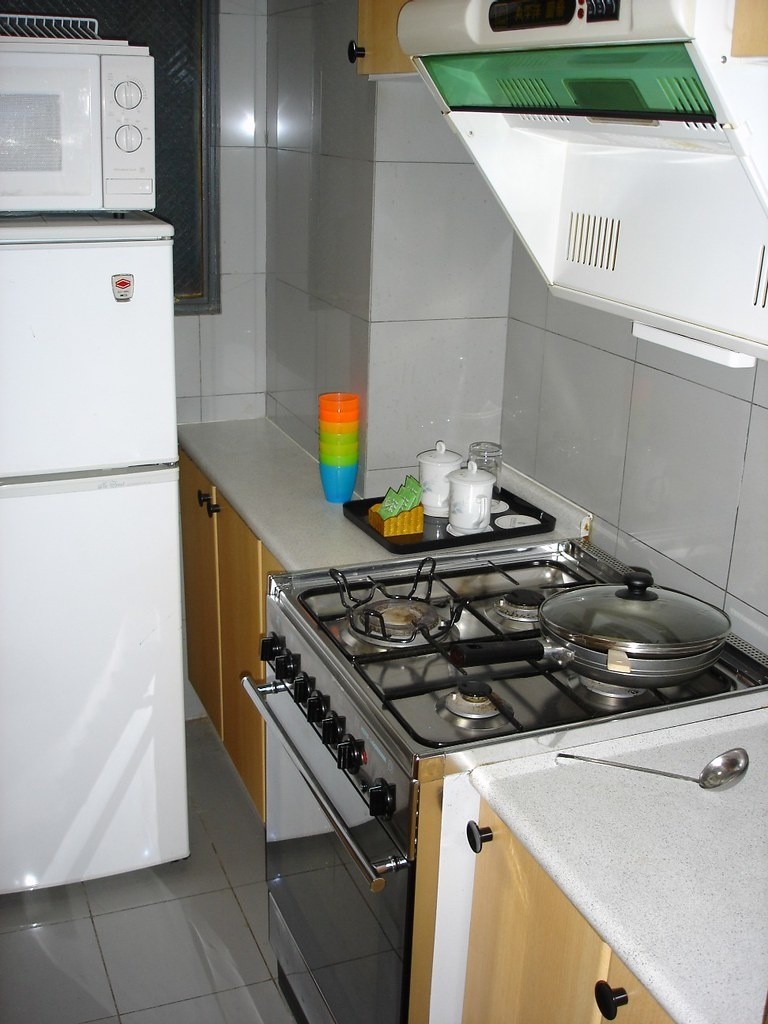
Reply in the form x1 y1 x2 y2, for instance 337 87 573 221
368 501 424 537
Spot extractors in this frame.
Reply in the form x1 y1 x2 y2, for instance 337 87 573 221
397 0 768 378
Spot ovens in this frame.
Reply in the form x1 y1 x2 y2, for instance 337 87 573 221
240 660 420 1024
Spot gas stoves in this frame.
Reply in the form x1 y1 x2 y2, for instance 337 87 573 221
270 534 768 775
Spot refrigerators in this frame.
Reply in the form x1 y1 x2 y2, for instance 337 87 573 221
0 211 188 895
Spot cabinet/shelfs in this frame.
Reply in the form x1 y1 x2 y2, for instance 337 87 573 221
460 798 679 1024
178 450 411 843
347 0 417 75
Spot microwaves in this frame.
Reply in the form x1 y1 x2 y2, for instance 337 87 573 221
0 38 156 218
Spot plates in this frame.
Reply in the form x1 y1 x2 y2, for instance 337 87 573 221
491 500 509 514
447 524 493 537
495 515 541 529
424 510 448 517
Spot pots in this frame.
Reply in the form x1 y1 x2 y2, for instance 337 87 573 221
451 572 733 688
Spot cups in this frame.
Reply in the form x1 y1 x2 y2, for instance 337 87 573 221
416 441 464 513
317 393 358 503
446 462 496 534
469 441 503 506
